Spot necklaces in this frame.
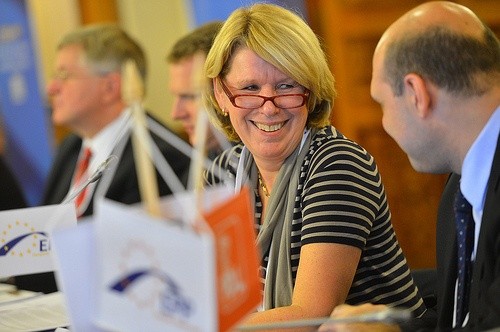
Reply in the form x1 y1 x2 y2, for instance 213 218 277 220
256 169 274 199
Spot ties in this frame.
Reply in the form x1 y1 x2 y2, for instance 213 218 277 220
453 181 476 330
72 146 92 222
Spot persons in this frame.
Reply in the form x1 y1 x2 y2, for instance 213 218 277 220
317 0 500 332
0 22 234 294
201 2 427 332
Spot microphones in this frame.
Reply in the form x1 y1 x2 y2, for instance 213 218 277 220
230 311 412 332
63 155 118 204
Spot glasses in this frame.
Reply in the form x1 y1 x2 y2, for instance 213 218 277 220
216 74 310 110
